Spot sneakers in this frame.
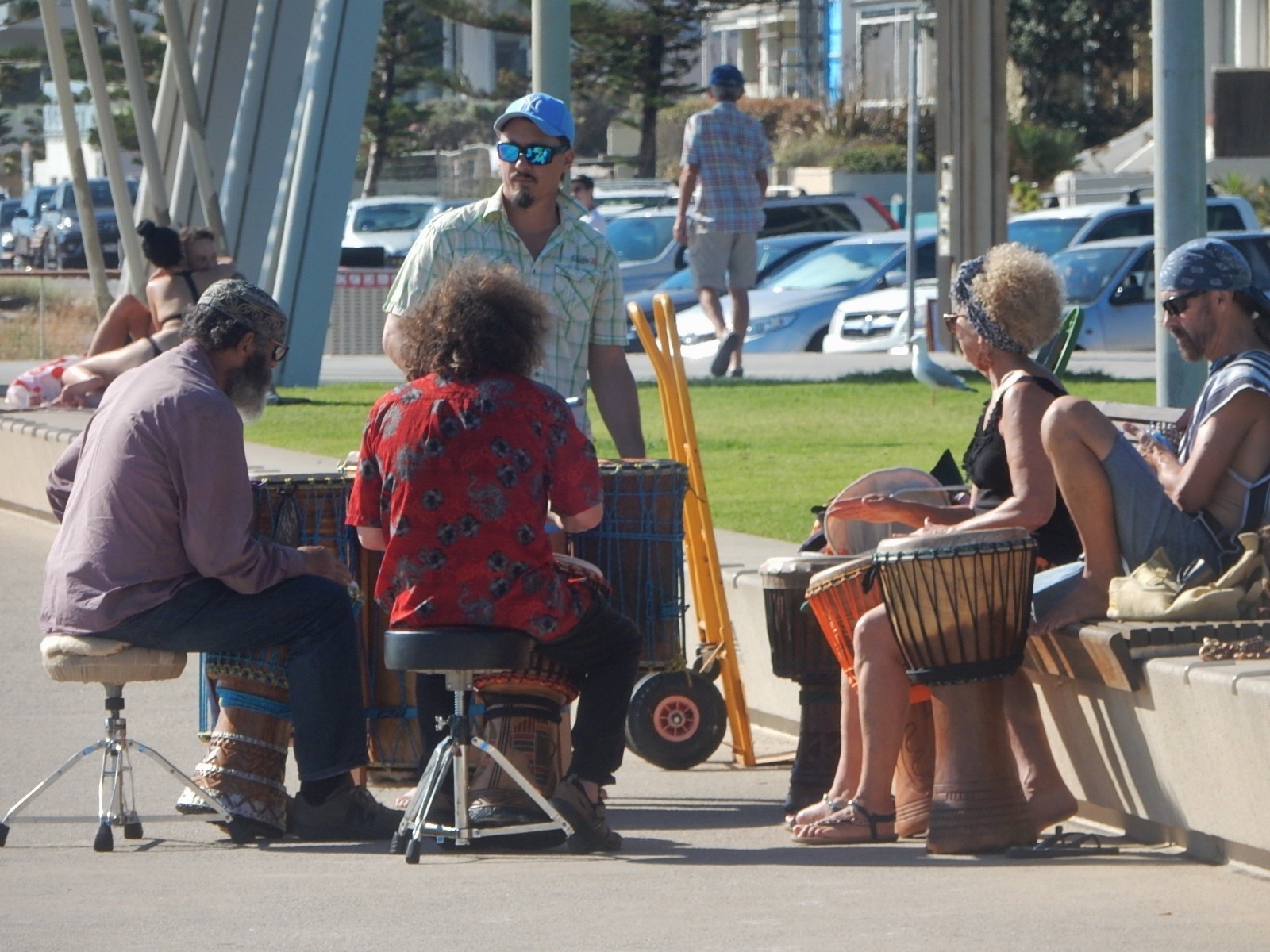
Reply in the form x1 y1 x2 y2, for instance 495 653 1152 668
550 775 623 847
294 778 411 841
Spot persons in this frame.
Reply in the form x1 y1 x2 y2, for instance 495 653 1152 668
1028 239 1270 636
570 175 598 211
41 280 406 840
11 202 61 240
347 261 643 853
381 92 646 463
783 239 1081 843
50 218 246 409
674 64 776 379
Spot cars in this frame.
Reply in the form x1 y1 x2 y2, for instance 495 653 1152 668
655 226 938 352
0 177 143 271
1048 231 1269 354
341 193 484 259
624 231 863 353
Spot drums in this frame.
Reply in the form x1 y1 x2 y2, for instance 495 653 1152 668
568 458 689 673
339 451 422 780
758 554 865 814
871 525 1038 854
250 470 345 557
174 641 293 839
466 551 614 854
804 555 936 838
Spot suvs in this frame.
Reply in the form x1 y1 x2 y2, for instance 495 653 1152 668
820 182 1261 356
603 183 901 291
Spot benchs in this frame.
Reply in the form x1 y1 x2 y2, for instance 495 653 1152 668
1011 399 1269 693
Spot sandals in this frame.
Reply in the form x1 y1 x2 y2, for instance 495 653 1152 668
790 796 898 844
783 791 848 832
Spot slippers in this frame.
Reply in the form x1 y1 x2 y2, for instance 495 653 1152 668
1004 822 1120 858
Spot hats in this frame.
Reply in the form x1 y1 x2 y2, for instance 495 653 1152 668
709 65 744 87
494 92 575 142
198 278 287 344
570 175 594 187
1159 238 1252 291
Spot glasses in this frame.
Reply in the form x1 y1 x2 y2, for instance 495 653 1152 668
570 186 586 193
495 139 571 167
266 336 290 359
1161 287 1201 315
941 310 974 331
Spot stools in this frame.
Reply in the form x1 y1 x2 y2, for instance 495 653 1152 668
0 634 249 853
382 627 589 864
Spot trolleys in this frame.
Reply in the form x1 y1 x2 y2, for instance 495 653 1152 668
196 293 758 771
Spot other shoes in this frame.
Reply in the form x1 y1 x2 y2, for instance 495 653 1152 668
419 792 455 828
710 333 744 379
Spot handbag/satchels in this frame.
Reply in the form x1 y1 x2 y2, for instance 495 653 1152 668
1106 528 1268 620
